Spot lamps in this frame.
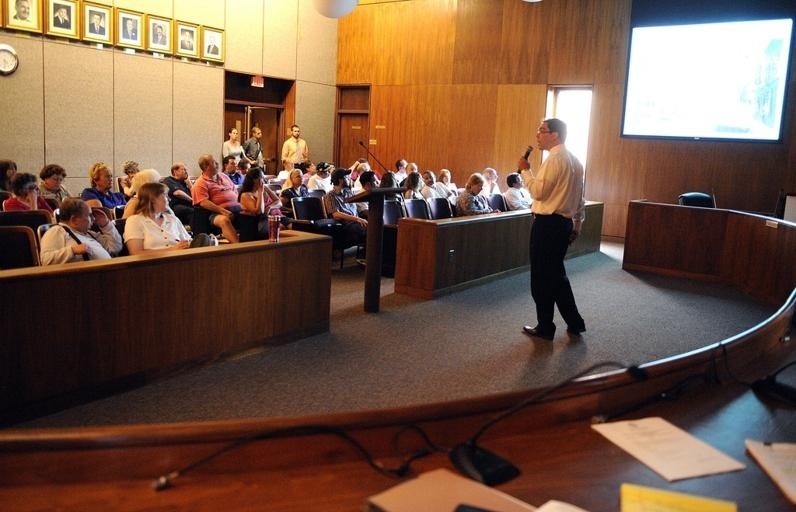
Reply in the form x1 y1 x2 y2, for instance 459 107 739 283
311 0 358 19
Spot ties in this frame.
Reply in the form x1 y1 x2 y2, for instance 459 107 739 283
296 141 302 165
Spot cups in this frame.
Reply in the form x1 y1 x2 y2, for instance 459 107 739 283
268 215 280 243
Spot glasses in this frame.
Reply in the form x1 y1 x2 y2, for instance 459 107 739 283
537 128 553 134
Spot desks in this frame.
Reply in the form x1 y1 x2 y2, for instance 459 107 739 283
0 229 333 430
394 200 605 301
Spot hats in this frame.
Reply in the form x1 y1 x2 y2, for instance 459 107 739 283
315 161 332 172
329 167 352 181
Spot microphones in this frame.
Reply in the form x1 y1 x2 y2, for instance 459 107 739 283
447 358 649 490
359 141 399 187
516 145 534 174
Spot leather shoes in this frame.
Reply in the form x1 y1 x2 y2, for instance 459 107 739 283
523 320 556 341
565 315 587 334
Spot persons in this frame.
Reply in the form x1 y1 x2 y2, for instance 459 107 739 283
325 169 369 264
206 34 219 55
152 25 166 44
456 167 533 217
53 7 70 29
0 153 283 267
181 31 192 51
223 127 242 164
240 127 262 167
516 117 587 339
89 12 104 36
10 0 30 22
122 19 137 40
279 160 457 212
280 124 309 176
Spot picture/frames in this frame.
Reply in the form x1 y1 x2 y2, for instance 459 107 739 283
0 0 226 64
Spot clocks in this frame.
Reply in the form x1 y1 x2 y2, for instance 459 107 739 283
0 43 19 76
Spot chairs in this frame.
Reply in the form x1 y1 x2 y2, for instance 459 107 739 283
678 191 717 208
